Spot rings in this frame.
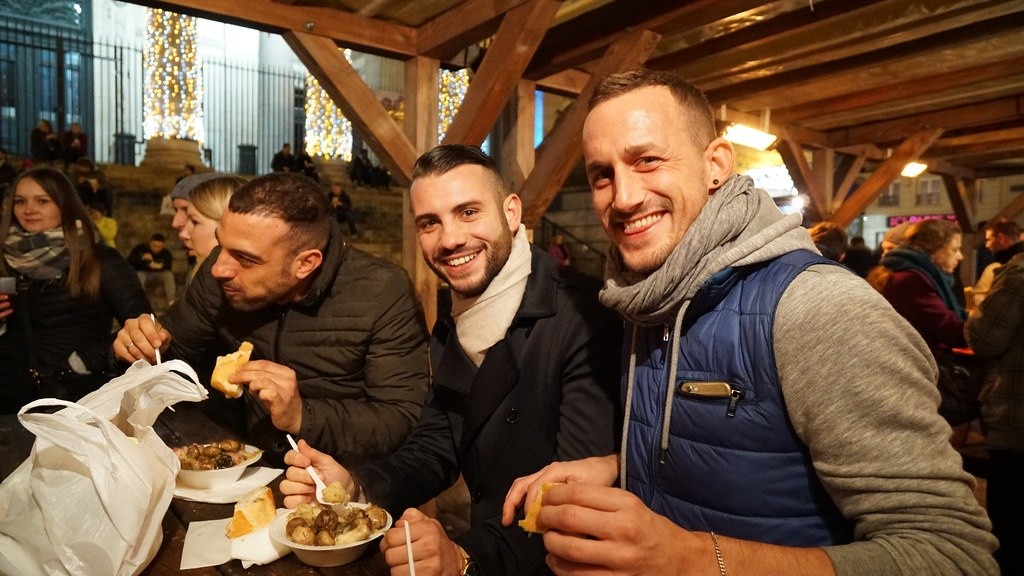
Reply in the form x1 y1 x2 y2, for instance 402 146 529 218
127 342 133 349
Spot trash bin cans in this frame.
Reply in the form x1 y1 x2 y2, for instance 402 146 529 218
237 145 257 175
114 134 135 166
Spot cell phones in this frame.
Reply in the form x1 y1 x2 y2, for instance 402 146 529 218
681 382 732 396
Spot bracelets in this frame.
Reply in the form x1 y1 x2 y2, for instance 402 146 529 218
710 532 725 576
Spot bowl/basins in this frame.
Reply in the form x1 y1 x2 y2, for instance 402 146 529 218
270 502 393 567
176 443 261 489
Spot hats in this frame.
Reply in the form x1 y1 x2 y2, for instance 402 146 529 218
171 171 236 201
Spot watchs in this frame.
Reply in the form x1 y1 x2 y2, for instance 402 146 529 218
459 547 478 576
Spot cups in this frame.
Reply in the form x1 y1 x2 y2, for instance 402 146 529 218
0 277 16 318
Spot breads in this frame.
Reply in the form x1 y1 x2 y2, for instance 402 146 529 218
518 482 564 534
210 341 254 399
226 486 278 539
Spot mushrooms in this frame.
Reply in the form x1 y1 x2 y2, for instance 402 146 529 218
286 504 388 547
179 438 245 471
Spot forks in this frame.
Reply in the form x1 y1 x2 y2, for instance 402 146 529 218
150 313 175 412
285 434 341 505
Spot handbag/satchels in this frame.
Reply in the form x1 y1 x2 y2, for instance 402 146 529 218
0 359 210 576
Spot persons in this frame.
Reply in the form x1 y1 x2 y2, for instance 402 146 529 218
0 166 157 419
128 233 173 306
501 67 1003 576
349 150 389 189
30 119 89 165
179 177 247 289
327 182 357 238
72 159 116 248
113 171 430 463
280 143 616 576
547 234 571 267
273 143 319 183
172 172 227 230
0 149 16 208
808 216 1024 576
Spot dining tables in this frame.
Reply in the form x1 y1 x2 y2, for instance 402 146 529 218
0 363 435 576
